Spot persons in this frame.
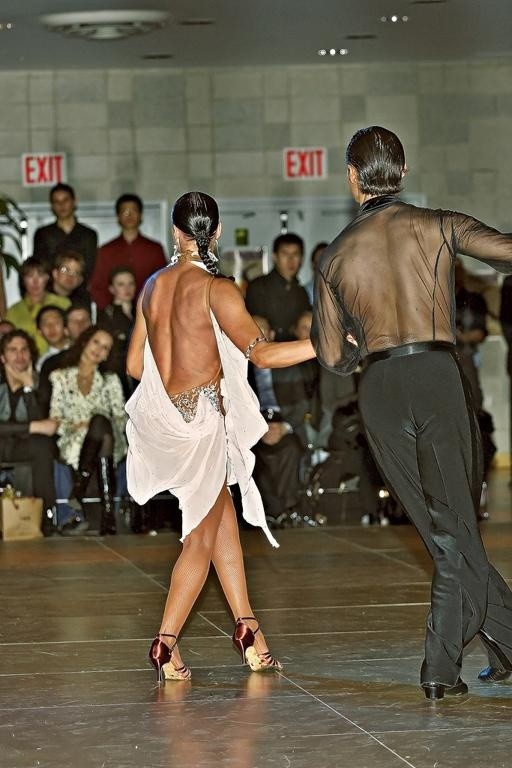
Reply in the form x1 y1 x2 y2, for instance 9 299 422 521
123 192 357 683
315 125 512 701
1 183 511 537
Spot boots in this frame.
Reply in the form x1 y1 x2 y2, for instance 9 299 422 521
61 437 104 537
96 456 117 536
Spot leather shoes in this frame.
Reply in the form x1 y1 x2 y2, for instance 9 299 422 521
478 665 512 682
422 676 468 700
41 503 58 536
274 514 299 527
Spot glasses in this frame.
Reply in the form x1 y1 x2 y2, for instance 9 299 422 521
60 266 83 277
121 209 137 214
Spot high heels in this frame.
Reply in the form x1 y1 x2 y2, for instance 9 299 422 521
232 617 283 672
149 634 191 683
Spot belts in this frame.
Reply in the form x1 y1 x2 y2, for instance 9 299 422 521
365 340 457 363
